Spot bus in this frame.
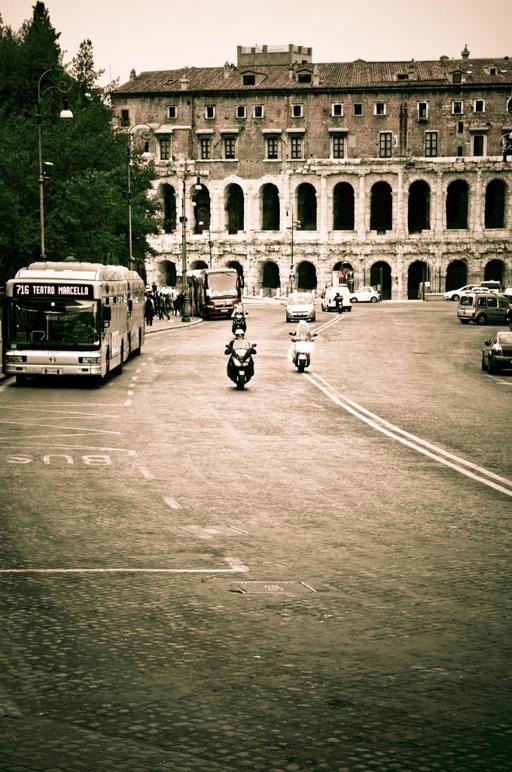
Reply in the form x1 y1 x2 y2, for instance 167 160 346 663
2 261 146 388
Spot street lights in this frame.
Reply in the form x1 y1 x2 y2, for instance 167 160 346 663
284 201 294 292
34 67 74 261
198 207 212 269
181 162 202 288
127 122 154 271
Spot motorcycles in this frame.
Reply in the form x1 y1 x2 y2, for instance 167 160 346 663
225 344 257 389
289 332 317 372
232 312 248 338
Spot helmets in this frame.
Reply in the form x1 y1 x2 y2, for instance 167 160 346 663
235 329 244 339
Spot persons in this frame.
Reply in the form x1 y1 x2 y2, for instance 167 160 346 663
144 291 184 326
292 320 314 343
506 302 512 331
152 282 157 293
226 329 256 355
334 293 344 311
72 318 98 339
231 301 249 318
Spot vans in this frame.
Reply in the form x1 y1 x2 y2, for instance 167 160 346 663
444 280 512 325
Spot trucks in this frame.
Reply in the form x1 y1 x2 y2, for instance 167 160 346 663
176 267 244 320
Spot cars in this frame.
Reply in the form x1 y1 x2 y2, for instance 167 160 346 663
281 283 381 322
482 330 512 375
